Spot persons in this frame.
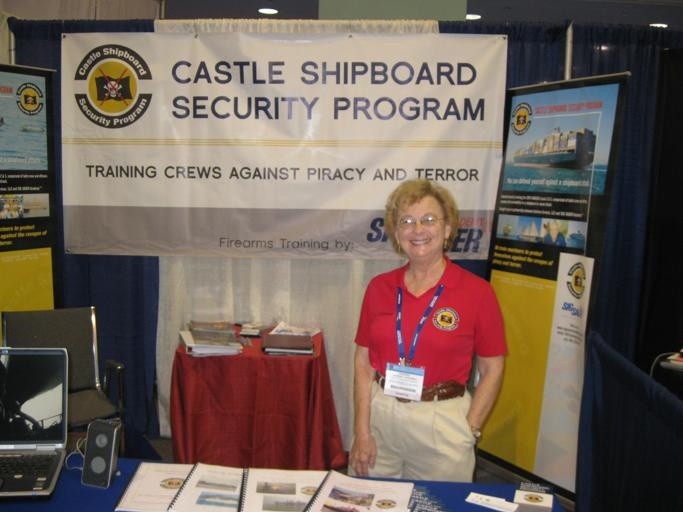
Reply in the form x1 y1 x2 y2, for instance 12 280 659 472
350 178 508 482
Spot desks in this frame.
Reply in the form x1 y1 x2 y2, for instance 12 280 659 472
2 453 564 511
173 327 343 466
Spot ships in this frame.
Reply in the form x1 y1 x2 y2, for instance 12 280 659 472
514 127 596 167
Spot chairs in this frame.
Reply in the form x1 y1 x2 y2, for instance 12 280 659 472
2 305 128 461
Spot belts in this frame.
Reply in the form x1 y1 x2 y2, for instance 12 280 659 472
375 370 464 404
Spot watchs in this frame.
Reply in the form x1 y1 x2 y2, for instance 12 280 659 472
471 428 483 438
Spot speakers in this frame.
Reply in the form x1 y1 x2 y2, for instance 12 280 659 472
81 419 122 489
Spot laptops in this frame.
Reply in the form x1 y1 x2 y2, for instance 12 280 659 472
0 347 69 498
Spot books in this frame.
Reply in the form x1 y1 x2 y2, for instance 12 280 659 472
114 456 414 512
178 315 321 358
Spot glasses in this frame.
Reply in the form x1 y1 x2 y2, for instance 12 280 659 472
396 214 445 230
